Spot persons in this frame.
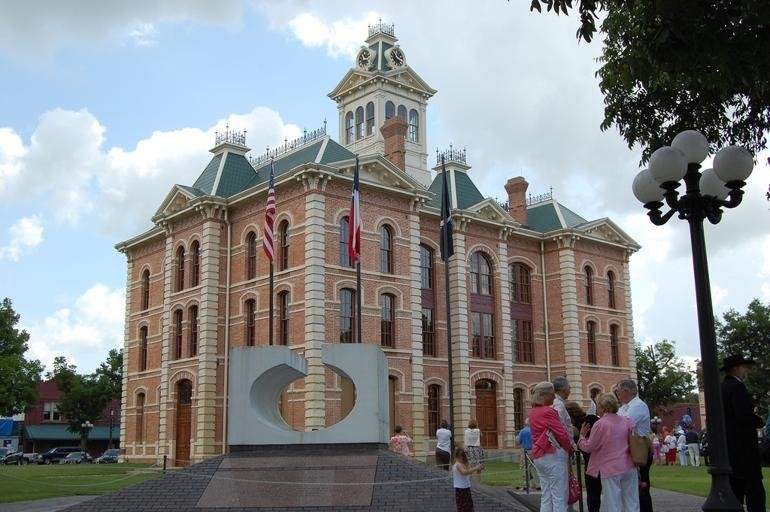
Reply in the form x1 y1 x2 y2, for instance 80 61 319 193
515 417 541 491
719 353 766 512
464 420 486 484
390 426 412 456
436 420 453 471
528 376 710 512
452 448 483 512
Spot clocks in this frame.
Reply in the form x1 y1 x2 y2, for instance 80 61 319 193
390 47 405 68
356 47 371 70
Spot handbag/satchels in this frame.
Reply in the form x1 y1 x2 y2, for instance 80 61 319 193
661 447 669 452
629 429 649 465
677 446 681 451
568 475 581 504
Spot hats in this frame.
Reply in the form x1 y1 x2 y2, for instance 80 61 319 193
678 429 686 435
720 354 756 371
532 381 554 394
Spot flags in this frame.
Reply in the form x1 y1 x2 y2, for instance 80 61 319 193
439 162 455 260
348 160 362 262
263 164 275 262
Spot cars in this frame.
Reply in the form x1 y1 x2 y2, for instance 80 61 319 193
0 446 120 467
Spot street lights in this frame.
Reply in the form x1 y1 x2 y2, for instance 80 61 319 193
81 420 93 456
650 415 662 435
630 129 754 512
109 408 114 449
